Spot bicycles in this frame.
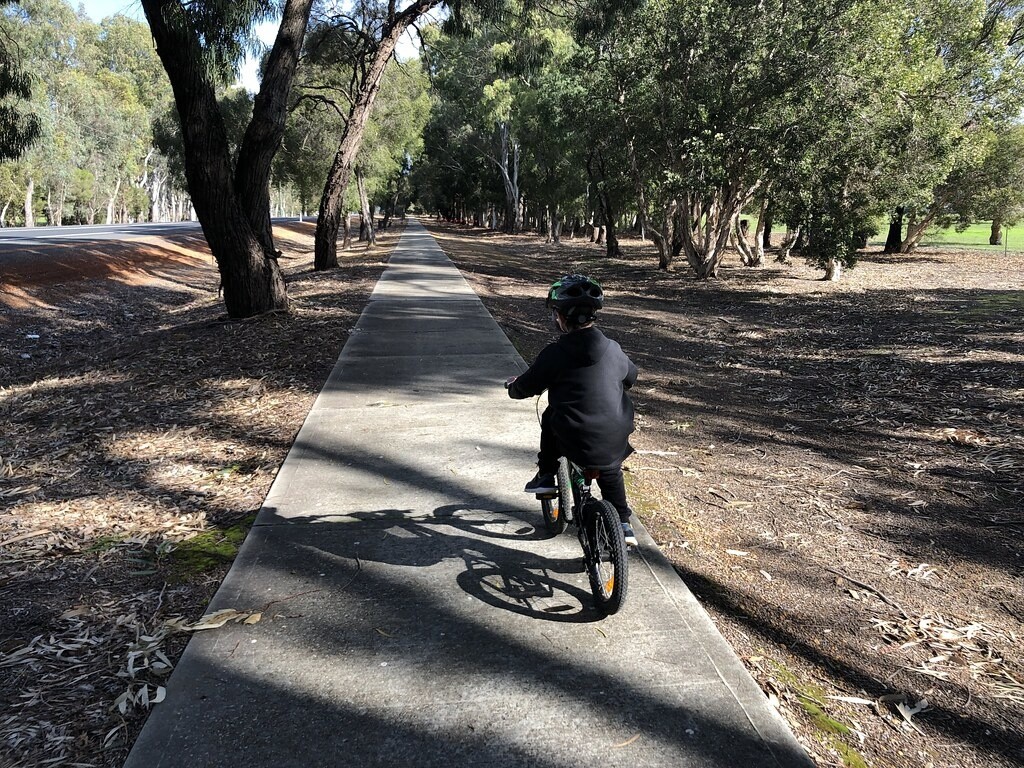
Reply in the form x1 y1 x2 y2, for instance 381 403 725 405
504 381 632 616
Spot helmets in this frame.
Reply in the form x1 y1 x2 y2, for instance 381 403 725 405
548 273 604 311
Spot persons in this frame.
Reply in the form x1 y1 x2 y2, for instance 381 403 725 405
506 274 638 545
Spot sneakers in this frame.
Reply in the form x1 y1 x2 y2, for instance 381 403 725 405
525 472 557 494
624 529 639 547
